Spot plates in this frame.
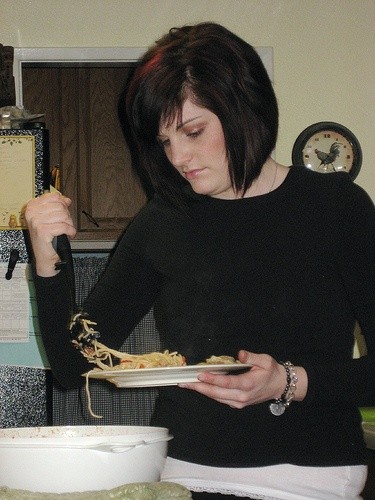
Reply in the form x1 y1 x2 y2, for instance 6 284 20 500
81 364 253 388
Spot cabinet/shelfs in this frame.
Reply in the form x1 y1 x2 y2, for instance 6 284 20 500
22 62 154 241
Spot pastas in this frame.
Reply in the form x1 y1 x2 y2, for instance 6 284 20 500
75 329 237 418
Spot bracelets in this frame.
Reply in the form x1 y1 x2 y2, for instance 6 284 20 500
270 363 298 416
276 362 291 403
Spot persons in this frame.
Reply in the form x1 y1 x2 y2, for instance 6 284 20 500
25 22 375 500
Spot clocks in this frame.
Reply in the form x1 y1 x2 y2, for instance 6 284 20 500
291 122 363 183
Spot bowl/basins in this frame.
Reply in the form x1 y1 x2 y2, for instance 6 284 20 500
0 425 173 494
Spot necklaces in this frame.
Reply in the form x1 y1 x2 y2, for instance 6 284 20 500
270 164 281 193
